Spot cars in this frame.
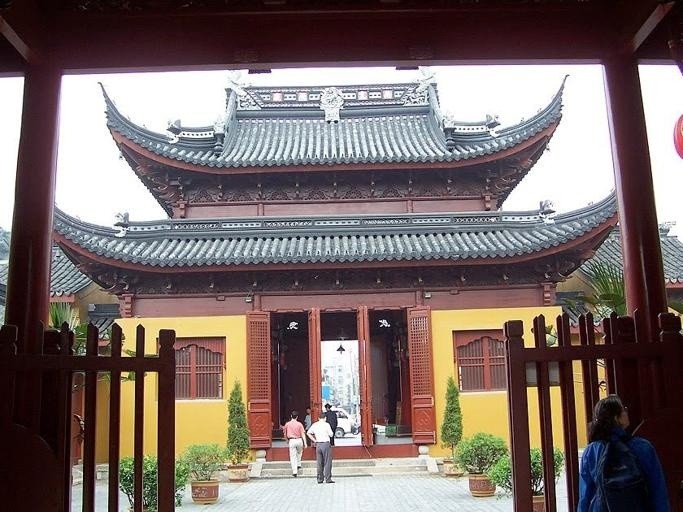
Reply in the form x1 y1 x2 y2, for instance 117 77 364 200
332 408 355 438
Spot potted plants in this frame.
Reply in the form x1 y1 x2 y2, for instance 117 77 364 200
179 377 251 504
438 374 564 511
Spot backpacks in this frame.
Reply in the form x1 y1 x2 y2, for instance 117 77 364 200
592 436 657 512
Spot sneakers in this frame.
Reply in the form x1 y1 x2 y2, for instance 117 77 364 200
293 466 335 483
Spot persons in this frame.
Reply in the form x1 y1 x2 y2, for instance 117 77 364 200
303 408 310 432
305 412 335 483
324 403 337 445
283 410 307 476
573 393 670 511
